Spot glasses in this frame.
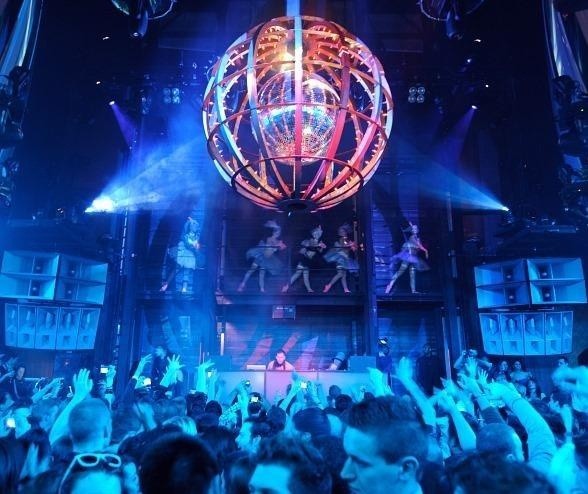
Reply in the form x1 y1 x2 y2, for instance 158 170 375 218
58 453 121 494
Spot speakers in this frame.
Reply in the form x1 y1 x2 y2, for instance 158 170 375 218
0 250 109 352
473 257 587 358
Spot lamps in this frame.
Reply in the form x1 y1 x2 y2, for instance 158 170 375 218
201 16 394 215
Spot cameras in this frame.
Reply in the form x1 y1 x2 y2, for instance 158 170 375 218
99 367 108 374
250 397 258 402
300 382 307 388
5 417 16 428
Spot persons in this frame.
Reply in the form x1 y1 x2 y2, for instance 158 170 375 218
235 219 287 295
283 224 328 293
0 347 588 494
155 217 214 294
384 223 428 293
321 221 360 294
